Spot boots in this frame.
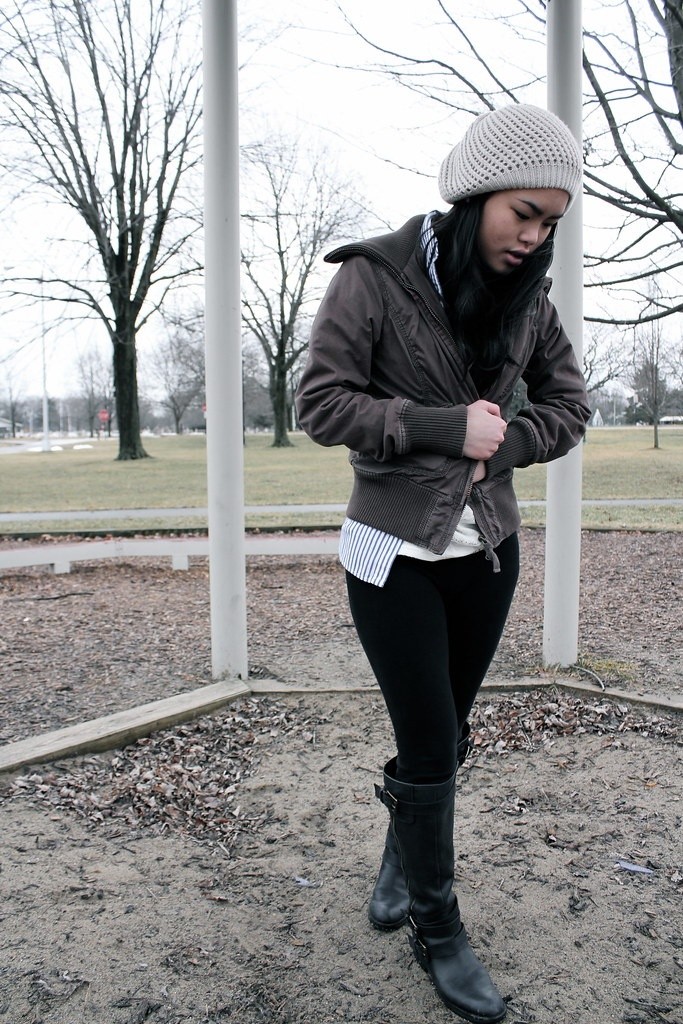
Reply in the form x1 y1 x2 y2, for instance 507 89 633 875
367 715 470 931
374 755 507 1022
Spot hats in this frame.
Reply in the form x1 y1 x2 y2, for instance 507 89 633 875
437 103 582 218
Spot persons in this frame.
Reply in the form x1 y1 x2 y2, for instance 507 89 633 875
294 104 592 1024
